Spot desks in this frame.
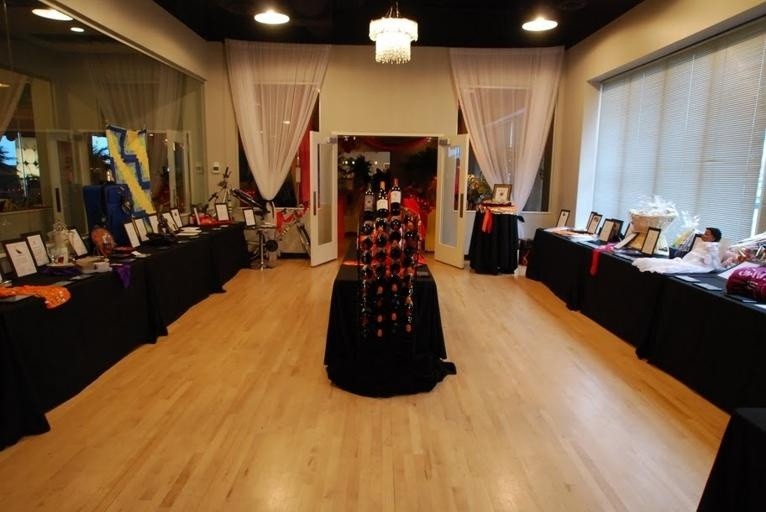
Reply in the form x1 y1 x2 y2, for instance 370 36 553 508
336 232 436 381
476 211 517 275
1 223 240 453
536 227 765 512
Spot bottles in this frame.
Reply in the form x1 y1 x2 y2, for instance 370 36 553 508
359 175 419 340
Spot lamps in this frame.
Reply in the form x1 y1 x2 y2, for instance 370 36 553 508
369 0 419 64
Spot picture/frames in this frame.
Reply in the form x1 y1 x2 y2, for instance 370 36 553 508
556 208 662 257
491 184 512 202
3 202 257 280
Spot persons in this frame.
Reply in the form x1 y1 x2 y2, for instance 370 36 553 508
702 227 722 245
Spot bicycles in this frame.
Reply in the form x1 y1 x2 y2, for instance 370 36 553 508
244 204 311 261
203 166 269 219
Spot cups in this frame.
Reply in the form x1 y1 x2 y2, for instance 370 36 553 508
95 261 110 272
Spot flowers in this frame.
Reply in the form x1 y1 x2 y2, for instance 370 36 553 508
467 174 491 204
